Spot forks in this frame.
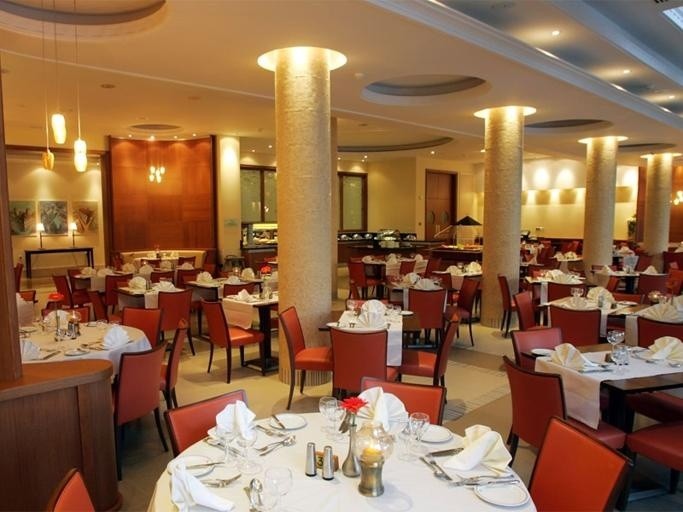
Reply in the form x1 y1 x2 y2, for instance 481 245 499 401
256 425 273 436
254 442 282 452
426 454 452 481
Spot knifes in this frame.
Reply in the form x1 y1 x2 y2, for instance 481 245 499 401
185 461 224 470
272 414 286 431
462 479 521 487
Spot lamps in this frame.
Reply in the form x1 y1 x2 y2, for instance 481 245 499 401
51 0 68 145
455 216 483 244
69 223 78 248
73 1 88 173
36 223 45 250
42 18 55 171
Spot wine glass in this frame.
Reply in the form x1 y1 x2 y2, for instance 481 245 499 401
320 396 336 430
409 413 430 452
621 345 632 375
392 306 401 321
613 330 625 349
96 319 107 332
326 399 345 440
611 347 625 376
233 422 262 475
386 304 393 317
606 331 618 353
346 299 358 318
216 417 235 464
265 466 291 512
263 287 272 301
252 479 277 512
398 421 417 460
346 299 357 311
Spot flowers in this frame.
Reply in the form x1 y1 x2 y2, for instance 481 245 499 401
337 396 368 434
48 292 63 318
260 265 272 281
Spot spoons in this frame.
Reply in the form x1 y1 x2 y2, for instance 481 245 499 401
419 456 447 483
250 478 264 505
259 434 296 457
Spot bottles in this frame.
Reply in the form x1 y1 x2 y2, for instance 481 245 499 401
305 442 316 476
323 445 334 480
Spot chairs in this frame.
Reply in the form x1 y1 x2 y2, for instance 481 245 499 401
666 267 682 296
121 306 163 350
44 467 97 511
398 320 459 404
439 277 481 347
527 414 634 512
111 252 126 314
223 282 256 298
158 327 189 411
158 285 196 357
150 270 175 286
178 256 197 266
591 265 620 292
628 386 682 495
603 293 647 332
141 258 162 269
431 271 461 338
662 251 683 275
348 279 361 300
549 304 602 347
547 281 588 302
404 288 447 350
62 305 90 323
348 260 386 300
87 290 123 325
51 273 90 308
68 269 91 308
199 296 267 384
163 389 250 458
103 273 134 315
112 337 170 482
175 268 205 288
14 255 39 304
636 316 683 350
496 273 532 338
636 273 672 307
420 257 443 278
633 254 654 271
501 355 626 468
399 259 417 276
506 326 610 447
359 376 447 429
328 325 399 402
279 305 340 410
512 289 551 331
615 418 683 511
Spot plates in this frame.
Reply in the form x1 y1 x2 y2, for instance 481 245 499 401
420 426 453 442
21 328 38 333
64 348 90 356
475 480 529 507
271 414 307 429
531 347 553 356
618 301 637 305
167 455 216 478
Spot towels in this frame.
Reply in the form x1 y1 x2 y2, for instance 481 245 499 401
162 262 172 270
414 253 423 261
648 336 683 364
387 258 397 264
416 278 434 289
237 289 250 300
128 277 146 288
139 266 154 274
645 303 680 323
182 263 194 270
360 300 386 312
43 310 69 326
362 255 372 263
226 276 240 283
240 268 255 279
549 343 597 373
403 273 419 282
153 281 176 291
123 263 136 272
99 323 128 349
445 266 460 273
15 293 27 304
209 401 256 443
196 272 212 284
466 261 481 272
388 254 396 258
565 252 572 258
358 313 386 328
355 386 408 436
98 268 112 277
80 267 96 276
546 269 575 283
18 338 40 361
170 463 234 512
443 424 513 476
588 287 613 306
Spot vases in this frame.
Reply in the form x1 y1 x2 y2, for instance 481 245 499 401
260 283 273 300
342 424 362 478
52 315 64 338
